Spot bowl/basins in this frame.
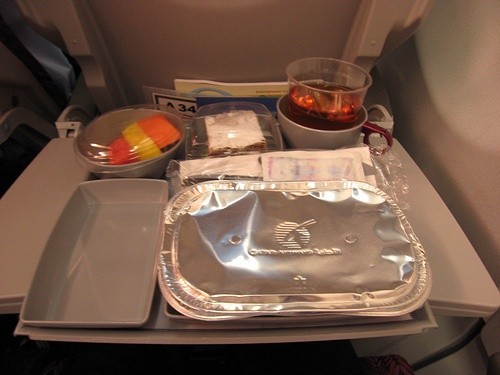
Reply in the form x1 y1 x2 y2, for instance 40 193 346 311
71 108 187 182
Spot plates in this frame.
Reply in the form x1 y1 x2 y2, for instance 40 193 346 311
157 179 432 322
184 113 283 160
19 178 169 329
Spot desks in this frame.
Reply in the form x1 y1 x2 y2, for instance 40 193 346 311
0 139 500 371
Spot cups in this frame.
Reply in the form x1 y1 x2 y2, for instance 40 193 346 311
284 57 372 131
276 92 393 155
